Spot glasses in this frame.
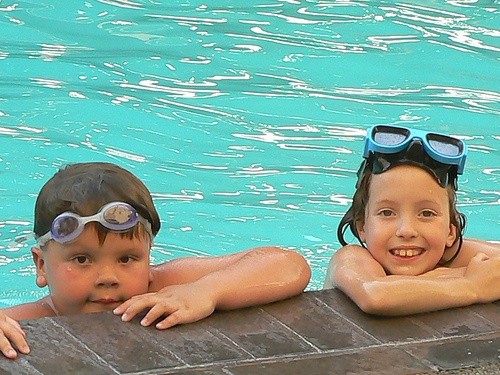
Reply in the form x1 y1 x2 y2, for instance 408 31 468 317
35 202 153 249
363 124 467 186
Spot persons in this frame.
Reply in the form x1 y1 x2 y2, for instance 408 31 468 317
323 131 499 318
0 162 312 360
